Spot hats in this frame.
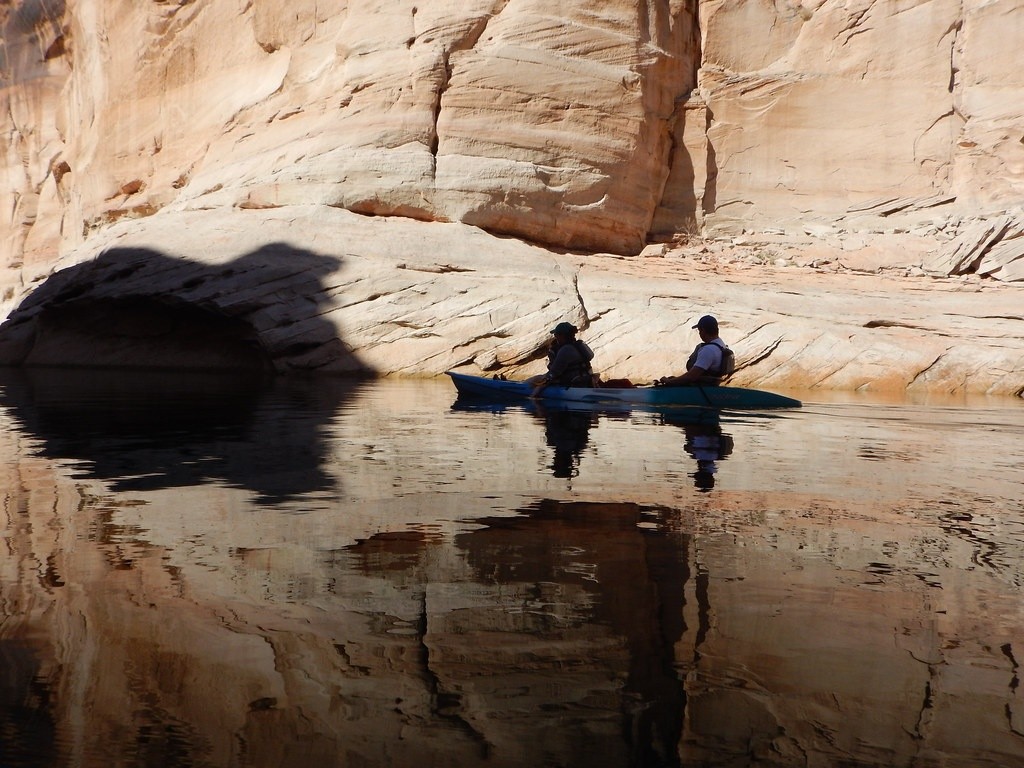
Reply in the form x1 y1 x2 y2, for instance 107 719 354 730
692 315 718 330
550 322 573 334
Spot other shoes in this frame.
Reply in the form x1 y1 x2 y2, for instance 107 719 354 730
596 378 607 388
493 375 500 380
501 373 507 381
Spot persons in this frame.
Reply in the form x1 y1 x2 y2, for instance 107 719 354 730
659 314 729 387
511 322 597 388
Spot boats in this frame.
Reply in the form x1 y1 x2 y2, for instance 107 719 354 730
442 370 804 411
